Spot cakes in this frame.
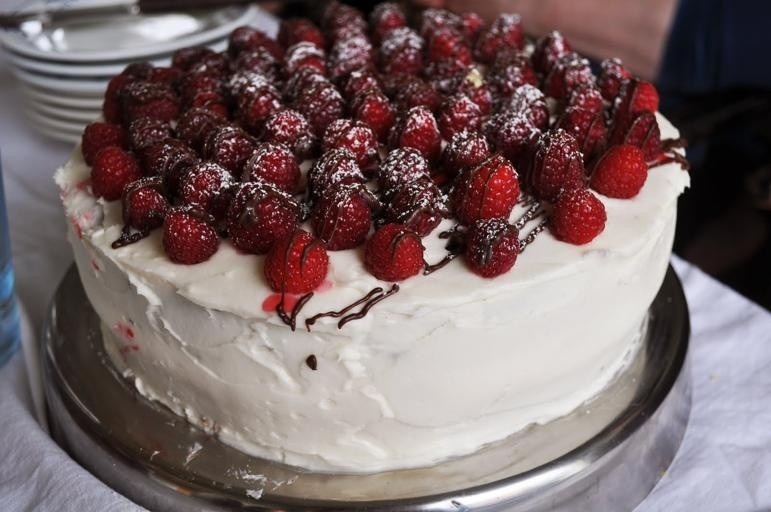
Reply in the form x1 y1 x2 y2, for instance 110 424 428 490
55 0 693 476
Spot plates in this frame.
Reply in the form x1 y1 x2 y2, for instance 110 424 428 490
1 1 276 146
